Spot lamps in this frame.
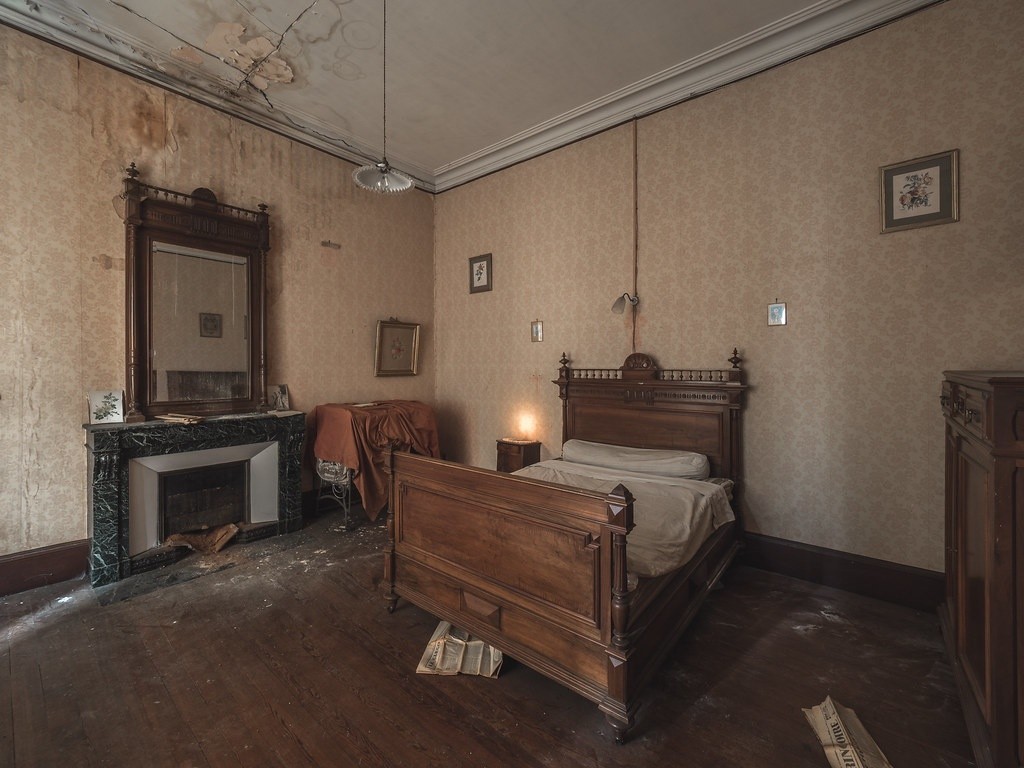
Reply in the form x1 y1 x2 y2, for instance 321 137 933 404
351 0 415 196
610 293 639 314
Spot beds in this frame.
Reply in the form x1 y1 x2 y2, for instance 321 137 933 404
383 347 751 743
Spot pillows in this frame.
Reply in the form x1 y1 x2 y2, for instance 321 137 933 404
561 439 709 480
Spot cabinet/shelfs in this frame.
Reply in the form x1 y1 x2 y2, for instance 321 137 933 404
939 367 1024 768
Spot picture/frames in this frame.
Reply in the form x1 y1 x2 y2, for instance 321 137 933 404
878 148 961 234
373 321 421 377
200 313 222 338
468 253 492 294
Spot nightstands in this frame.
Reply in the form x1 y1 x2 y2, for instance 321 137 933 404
495 437 542 473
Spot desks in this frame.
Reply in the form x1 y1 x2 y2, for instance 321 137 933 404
311 397 440 530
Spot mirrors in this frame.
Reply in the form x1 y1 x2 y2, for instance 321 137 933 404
120 162 271 424
